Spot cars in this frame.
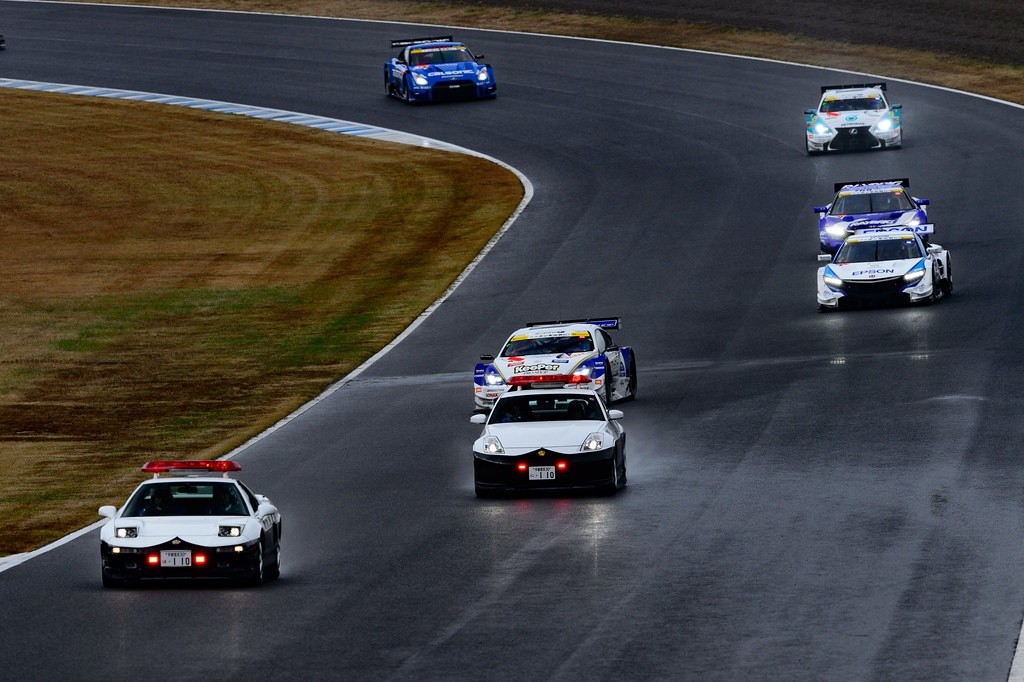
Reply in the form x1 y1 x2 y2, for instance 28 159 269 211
468 388 627 496
813 178 930 258
817 230 953 311
803 82 903 155
383 35 497 104
474 317 636 411
99 460 284 589
